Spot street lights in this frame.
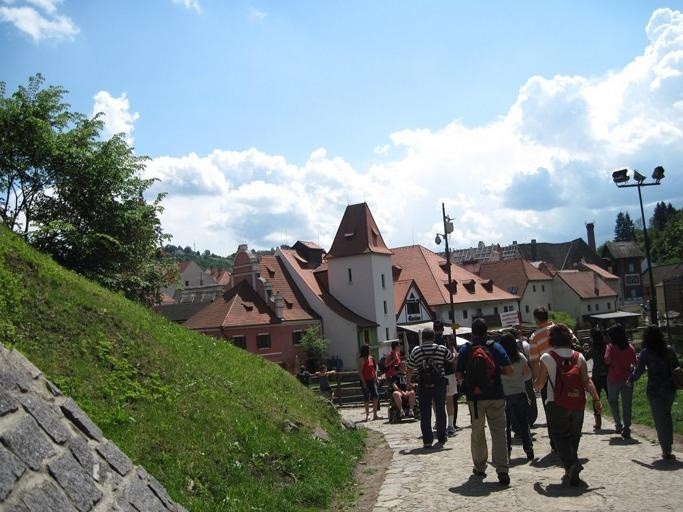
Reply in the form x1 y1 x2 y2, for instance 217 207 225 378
611 166 666 326
433 201 456 352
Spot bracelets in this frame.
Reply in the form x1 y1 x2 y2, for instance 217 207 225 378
592 398 601 403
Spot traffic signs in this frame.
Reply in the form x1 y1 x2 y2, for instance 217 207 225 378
500 311 519 329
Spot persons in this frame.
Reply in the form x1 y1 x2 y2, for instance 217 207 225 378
532 322 603 489
583 322 637 443
626 326 680 461
315 364 335 397
495 306 557 460
406 319 460 451
454 317 514 487
296 365 310 385
356 342 416 418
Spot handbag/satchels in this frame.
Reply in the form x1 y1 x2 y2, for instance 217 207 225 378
671 367 683 390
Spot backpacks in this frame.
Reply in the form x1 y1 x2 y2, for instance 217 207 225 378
464 340 502 392
417 344 442 385
547 350 586 409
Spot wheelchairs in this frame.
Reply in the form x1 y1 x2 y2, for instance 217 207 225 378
387 363 422 423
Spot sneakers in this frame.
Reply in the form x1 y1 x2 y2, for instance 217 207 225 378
400 410 405 416
424 423 462 448
496 471 510 486
615 423 631 439
409 410 414 416
524 446 534 459
